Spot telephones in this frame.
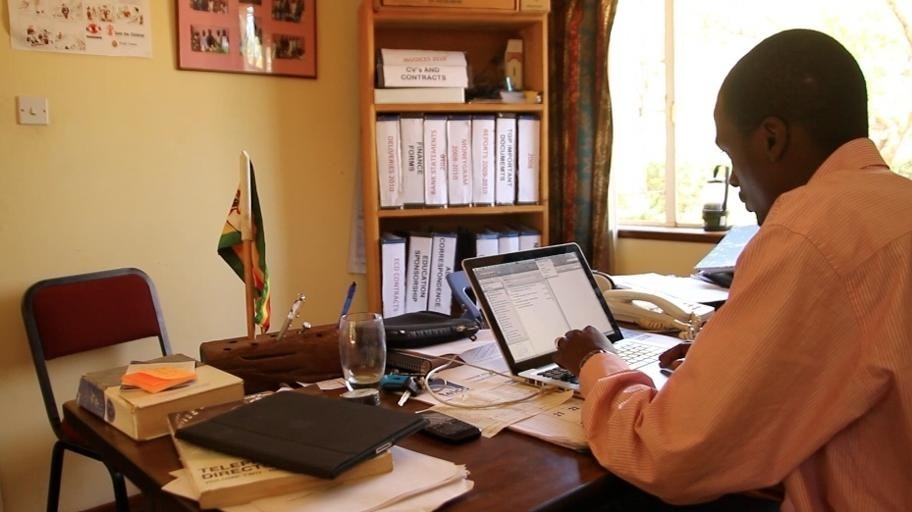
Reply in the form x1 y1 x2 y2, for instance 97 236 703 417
600 287 715 341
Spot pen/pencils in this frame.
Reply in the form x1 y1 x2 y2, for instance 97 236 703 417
277 292 305 337
337 283 358 323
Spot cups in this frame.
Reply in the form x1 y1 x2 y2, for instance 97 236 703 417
339 310 388 390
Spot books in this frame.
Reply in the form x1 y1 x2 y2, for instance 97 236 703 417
692 225 760 274
632 291 716 331
165 390 393 509
386 329 506 372
373 86 464 105
75 353 243 442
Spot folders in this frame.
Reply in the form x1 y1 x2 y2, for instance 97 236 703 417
175 390 430 478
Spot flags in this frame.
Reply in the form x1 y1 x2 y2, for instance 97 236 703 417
218 159 270 333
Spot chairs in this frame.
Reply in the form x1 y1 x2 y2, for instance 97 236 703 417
20 267 178 512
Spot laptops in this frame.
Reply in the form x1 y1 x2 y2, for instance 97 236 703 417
463 242 672 398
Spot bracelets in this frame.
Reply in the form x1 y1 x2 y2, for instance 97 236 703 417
576 350 613 380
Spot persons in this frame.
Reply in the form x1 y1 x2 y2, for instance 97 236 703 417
553 28 911 512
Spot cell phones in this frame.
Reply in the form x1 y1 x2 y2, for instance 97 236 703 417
417 409 480 445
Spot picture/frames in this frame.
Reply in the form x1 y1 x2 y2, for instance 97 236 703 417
360 0 550 316
174 3 318 82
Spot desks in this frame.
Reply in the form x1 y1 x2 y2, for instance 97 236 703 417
65 302 724 510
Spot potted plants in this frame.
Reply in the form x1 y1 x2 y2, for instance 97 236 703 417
701 164 730 231
